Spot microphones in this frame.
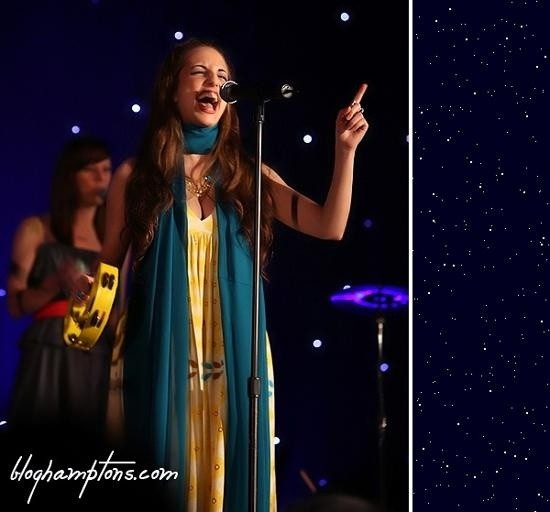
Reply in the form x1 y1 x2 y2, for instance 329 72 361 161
220 81 294 104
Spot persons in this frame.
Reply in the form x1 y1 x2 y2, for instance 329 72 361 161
6 141 118 432
72 37 370 512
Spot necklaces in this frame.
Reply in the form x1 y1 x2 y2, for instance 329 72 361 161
183 174 215 196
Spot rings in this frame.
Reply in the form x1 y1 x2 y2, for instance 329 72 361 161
77 291 85 298
359 108 365 113
